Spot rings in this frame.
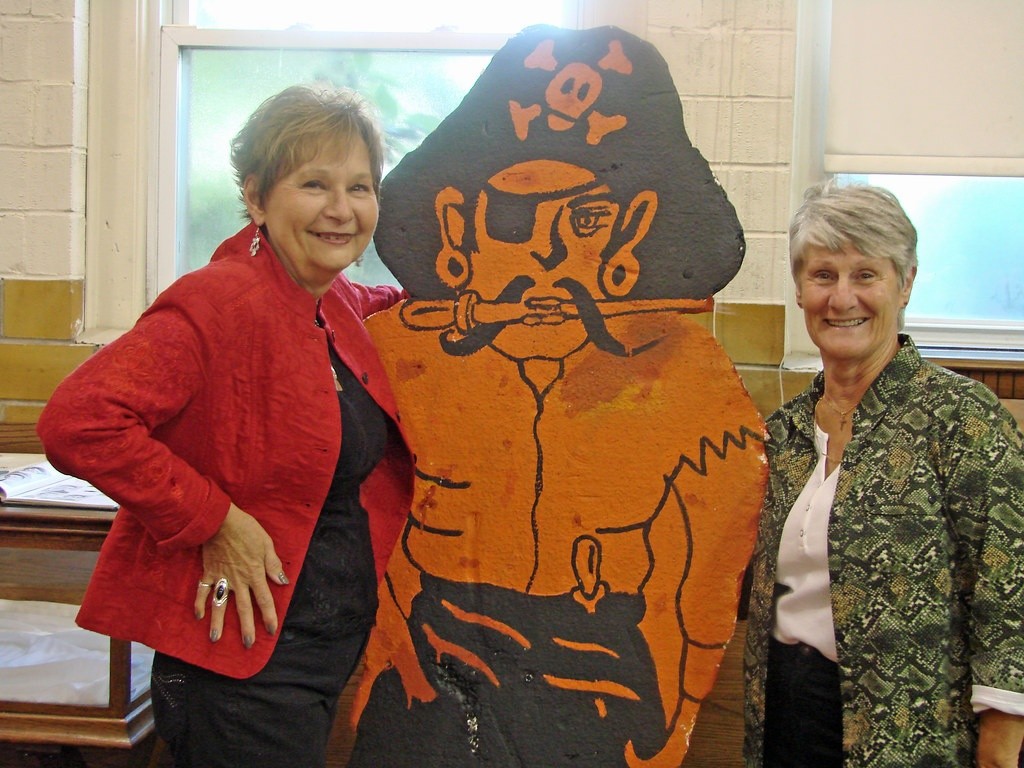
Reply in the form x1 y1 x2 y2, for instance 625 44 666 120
211 576 229 607
198 579 210 587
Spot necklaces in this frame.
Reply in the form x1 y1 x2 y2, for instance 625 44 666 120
822 395 862 430
814 400 845 463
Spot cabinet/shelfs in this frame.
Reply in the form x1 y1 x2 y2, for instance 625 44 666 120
0 452 154 746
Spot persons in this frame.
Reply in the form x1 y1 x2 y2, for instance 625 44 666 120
734 180 1024 768
34 85 418 767
